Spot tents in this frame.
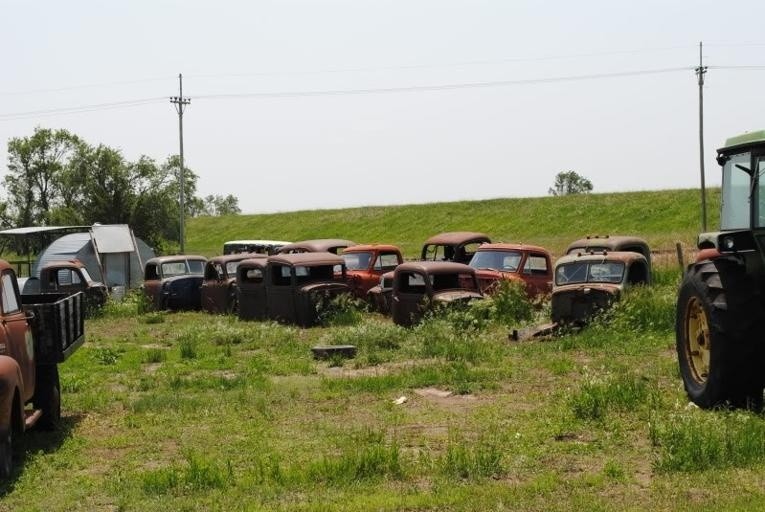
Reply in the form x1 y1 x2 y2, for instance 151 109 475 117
29 226 158 294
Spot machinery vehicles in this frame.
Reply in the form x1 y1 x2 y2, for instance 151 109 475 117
676 130 764 408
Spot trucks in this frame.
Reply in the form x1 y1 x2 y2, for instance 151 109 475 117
0 263 86 480
